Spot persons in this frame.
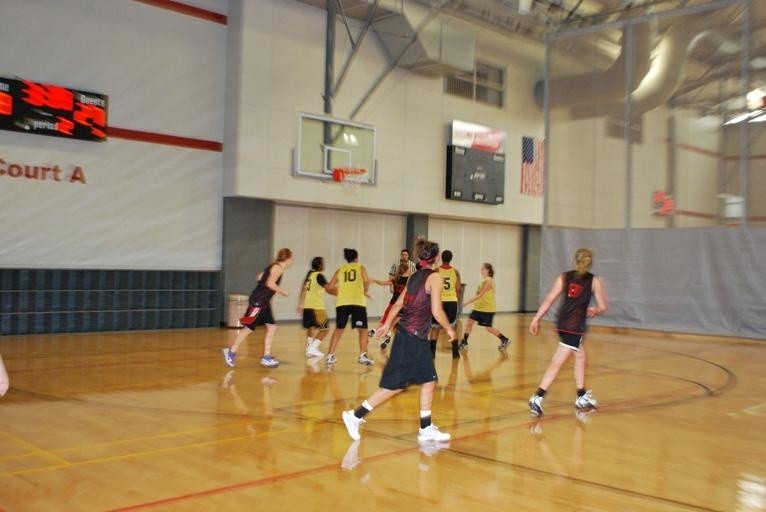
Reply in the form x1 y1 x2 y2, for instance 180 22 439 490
340 234 457 444
526 247 607 419
297 255 340 360
366 248 513 360
324 247 375 367
221 246 293 369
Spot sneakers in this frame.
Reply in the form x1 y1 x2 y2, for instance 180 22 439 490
260 358 280 368
418 423 451 442
222 348 235 368
528 392 544 416
574 389 599 410
342 409 366 441
368 328 391 350
430 337 511 359
305 345 376 367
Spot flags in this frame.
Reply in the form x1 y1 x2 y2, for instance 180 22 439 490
521 135 546 198
